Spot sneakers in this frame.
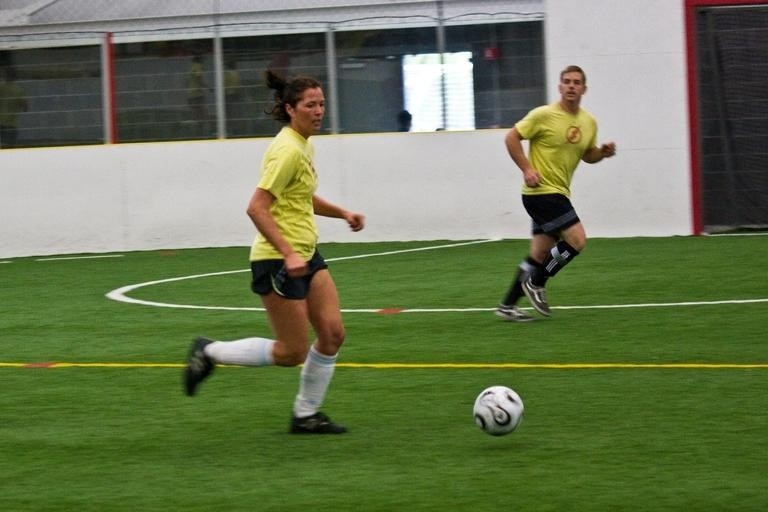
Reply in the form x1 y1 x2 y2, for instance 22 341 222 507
494 303 535 323
520 272 553 319
180 334 216 400
287 406 350 437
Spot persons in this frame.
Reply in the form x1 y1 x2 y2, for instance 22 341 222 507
0 62 31 147
181 64 366 438
391 110 414 131
491 63 616 322
254 35 295 132
181 48 213 136
224 59 242 133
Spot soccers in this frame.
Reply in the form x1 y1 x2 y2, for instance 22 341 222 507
472 384 527 436
